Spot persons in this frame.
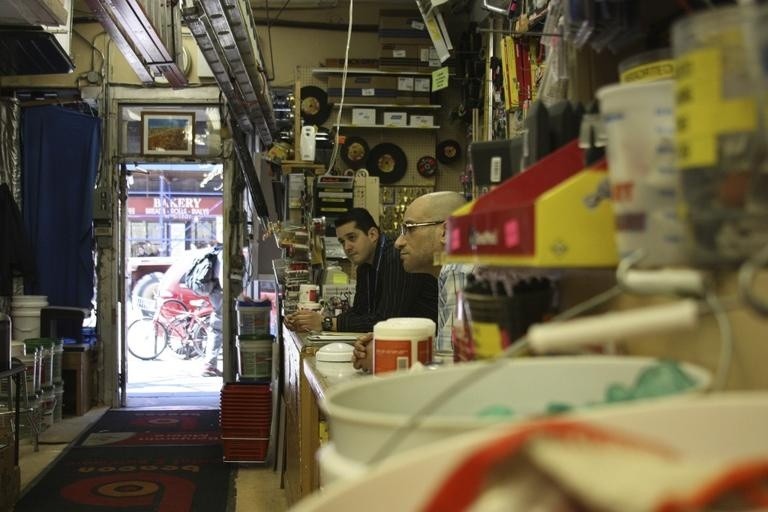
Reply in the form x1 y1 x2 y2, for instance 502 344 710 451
136 247 148 257
307 215 327 286
283 207 437 332
201 244 225 377
352 191 468 375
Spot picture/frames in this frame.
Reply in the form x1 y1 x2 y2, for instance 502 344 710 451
138 111 195 159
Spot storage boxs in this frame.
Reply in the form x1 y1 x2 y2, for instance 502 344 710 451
233 299 274 383
326 6 456 105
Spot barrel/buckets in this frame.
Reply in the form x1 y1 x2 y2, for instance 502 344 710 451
235 299 272 335
12 295 49 341
300 126 316 161
236 336 273 383
595 80 684 266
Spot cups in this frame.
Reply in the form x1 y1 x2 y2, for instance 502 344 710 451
596 5 768 268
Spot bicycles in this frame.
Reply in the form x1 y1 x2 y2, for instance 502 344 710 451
124 299 225 360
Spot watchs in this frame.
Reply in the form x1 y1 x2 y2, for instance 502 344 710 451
322 317 334 329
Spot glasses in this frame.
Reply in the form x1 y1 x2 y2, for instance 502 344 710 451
401 221 445 234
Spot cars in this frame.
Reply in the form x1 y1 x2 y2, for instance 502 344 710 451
154 249 225 337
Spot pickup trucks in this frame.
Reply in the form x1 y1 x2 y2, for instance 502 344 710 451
129 248 221 318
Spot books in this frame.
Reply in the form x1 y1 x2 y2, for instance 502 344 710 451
278 224 308 317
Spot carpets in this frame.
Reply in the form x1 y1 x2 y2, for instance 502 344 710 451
13 408 238 511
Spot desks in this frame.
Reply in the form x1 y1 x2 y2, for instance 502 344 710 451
277 320 359 504
63 342 93 417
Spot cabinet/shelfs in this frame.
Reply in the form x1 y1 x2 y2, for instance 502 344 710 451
311 65 456 131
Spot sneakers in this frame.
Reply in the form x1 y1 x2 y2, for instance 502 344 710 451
202 365 222 377
